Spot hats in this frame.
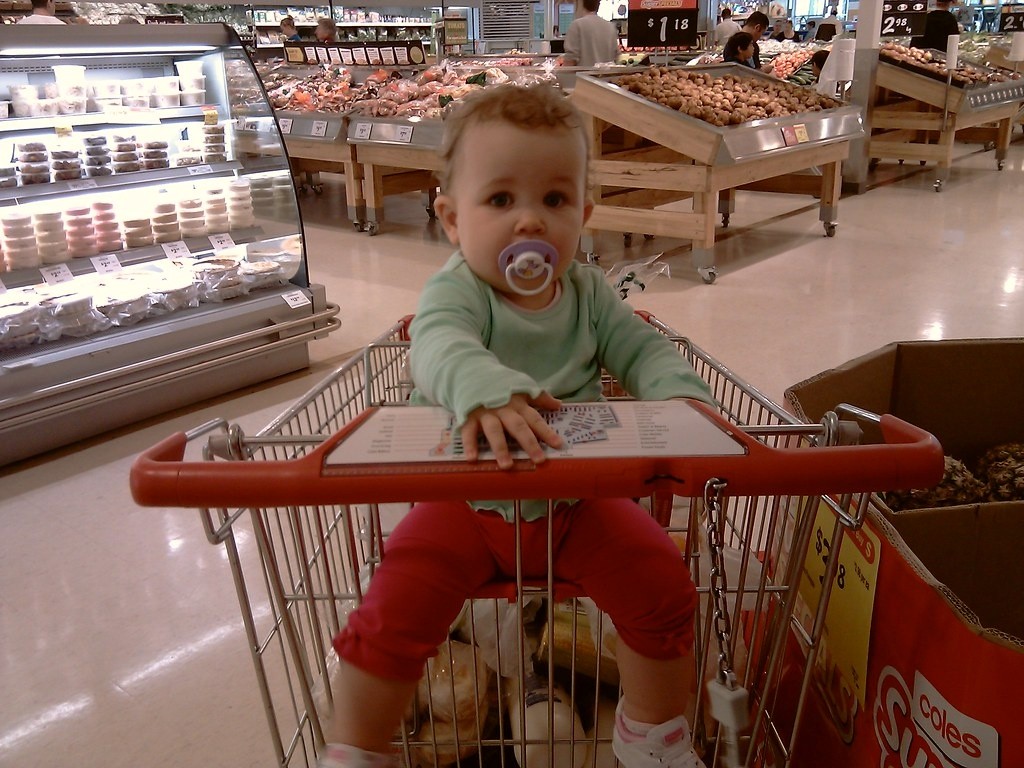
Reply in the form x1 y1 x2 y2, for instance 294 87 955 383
937 0 955 3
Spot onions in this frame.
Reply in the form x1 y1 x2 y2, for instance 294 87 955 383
880 42 1021 86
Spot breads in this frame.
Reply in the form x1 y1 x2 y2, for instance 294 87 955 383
393 602 510 765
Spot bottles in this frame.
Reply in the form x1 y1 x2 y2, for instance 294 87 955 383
800 16 806 31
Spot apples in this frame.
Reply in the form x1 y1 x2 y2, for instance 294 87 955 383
770 49 815 79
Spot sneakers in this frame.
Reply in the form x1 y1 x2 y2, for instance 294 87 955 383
612 695 709 768
318 744 401 768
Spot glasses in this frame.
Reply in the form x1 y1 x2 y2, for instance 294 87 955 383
786 21 792 23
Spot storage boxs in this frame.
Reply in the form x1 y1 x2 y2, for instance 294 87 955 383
765 337 1023 768
537 602 620 684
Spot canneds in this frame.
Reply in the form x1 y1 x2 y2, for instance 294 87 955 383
536 620 620 687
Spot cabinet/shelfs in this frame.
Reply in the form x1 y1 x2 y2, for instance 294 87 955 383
0 20 342 468
255 21 433 49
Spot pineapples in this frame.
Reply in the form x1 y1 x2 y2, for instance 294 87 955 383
877 440 1024 509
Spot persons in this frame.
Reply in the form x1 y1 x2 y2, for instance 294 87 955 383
806 49 830 86
714 8 742 47
553 24 561 38
726 32 757 69
280 15 302 42
15 0 67 24
314 17 337 43
312 83 718 768
723 11 774 74
909 0 961 52
765 6 843 43
562 0 618 67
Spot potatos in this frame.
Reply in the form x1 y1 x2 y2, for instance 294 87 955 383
607 66 839 127
352 67 556 120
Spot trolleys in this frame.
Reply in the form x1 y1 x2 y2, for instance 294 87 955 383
128 308 945 768
